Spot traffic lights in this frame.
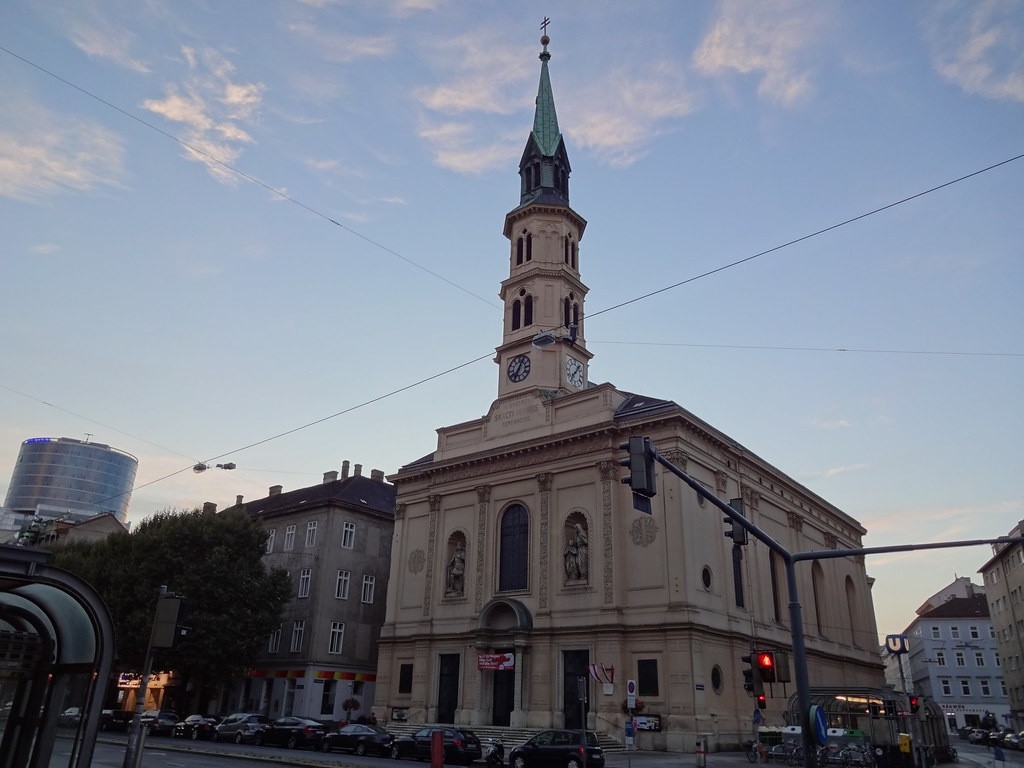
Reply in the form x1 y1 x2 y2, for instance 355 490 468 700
758 695 766 709
618 435 648 494
758 650 777 684
719 498 749 545
741 653 756 697
909 695 921 713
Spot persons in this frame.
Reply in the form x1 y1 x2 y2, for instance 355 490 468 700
981 730 989 745
563 523 588 580
368 712 377 725
448 542 465 590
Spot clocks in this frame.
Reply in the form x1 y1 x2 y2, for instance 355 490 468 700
566 354 585 387
508 354 531 383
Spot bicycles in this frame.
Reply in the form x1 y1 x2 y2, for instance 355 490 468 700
746 739 879 767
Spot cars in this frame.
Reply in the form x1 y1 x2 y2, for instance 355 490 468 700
959 726 1024 751
58 706 88 728
509 729 606 768
0 701 45 720
322 722 397 757
253 717 328 749
170 713 223 741
100 709 130 733
127 709 180 736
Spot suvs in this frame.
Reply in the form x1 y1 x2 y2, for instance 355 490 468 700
389 727 482 765
214 713 271 745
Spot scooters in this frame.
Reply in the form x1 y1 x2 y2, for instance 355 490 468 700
485 733 505 768
